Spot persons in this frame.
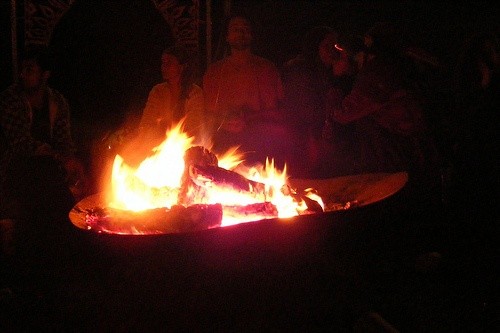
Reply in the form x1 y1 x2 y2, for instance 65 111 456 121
139 47 203 135
0 44 83 177
285 28 411 165
328 24 425 141
201 12 283 141
